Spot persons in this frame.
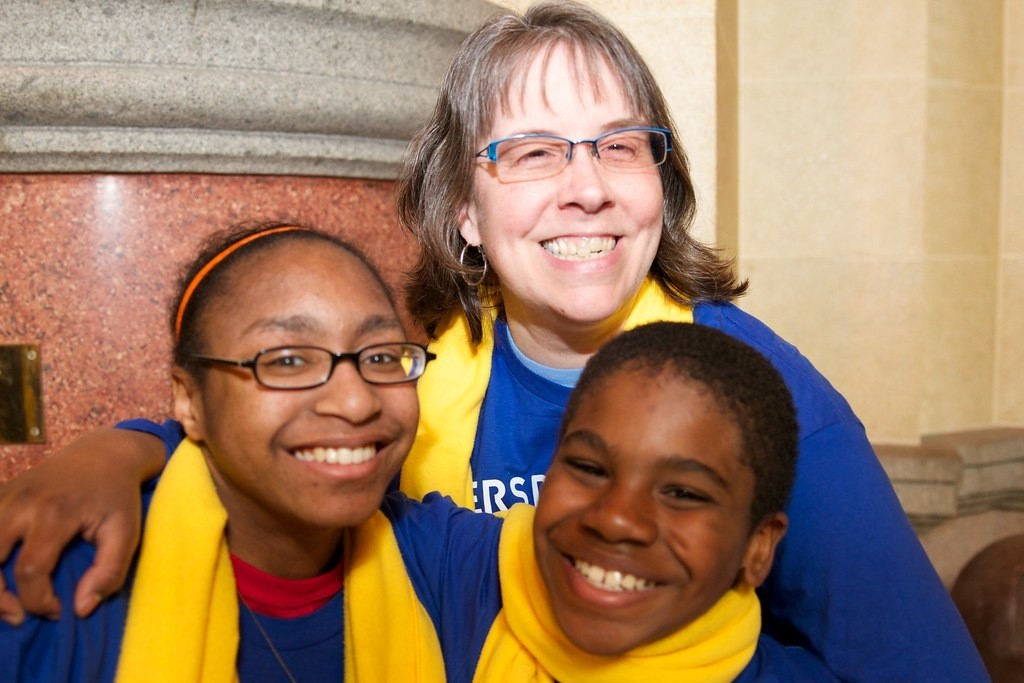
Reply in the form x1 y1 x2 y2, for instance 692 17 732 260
0 321 838 683
0 0 992 683
0 216 449 683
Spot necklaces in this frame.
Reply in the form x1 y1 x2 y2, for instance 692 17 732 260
235 586 298 683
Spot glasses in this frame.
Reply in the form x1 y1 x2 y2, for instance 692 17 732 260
466 126 673 183
184 343 436 391
